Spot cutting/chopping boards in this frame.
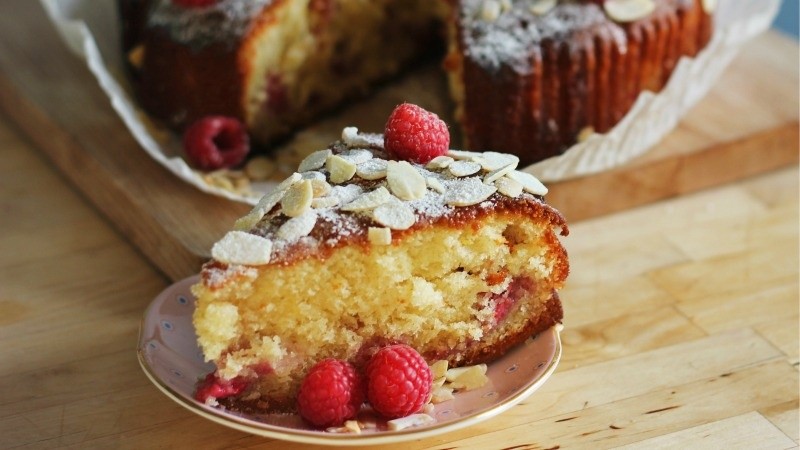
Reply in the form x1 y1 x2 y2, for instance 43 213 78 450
0 2 799 278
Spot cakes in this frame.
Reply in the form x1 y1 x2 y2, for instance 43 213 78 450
113 0 719 172
188 103 573 416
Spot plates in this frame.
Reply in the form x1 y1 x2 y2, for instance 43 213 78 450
136 274 561 445
